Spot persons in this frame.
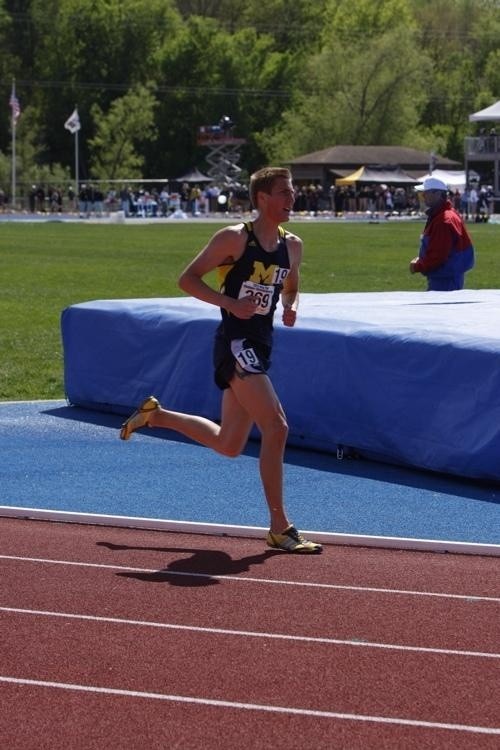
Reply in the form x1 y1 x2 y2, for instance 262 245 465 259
408 177 473 292
27 180 499 227
118 165 325 555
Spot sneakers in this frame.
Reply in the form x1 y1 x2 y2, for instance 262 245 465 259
120 396 162 440
266 524 323 554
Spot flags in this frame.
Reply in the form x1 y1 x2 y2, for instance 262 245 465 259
9 87 22 122
64 107 81 135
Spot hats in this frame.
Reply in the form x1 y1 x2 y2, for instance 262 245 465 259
414 178 448 192
